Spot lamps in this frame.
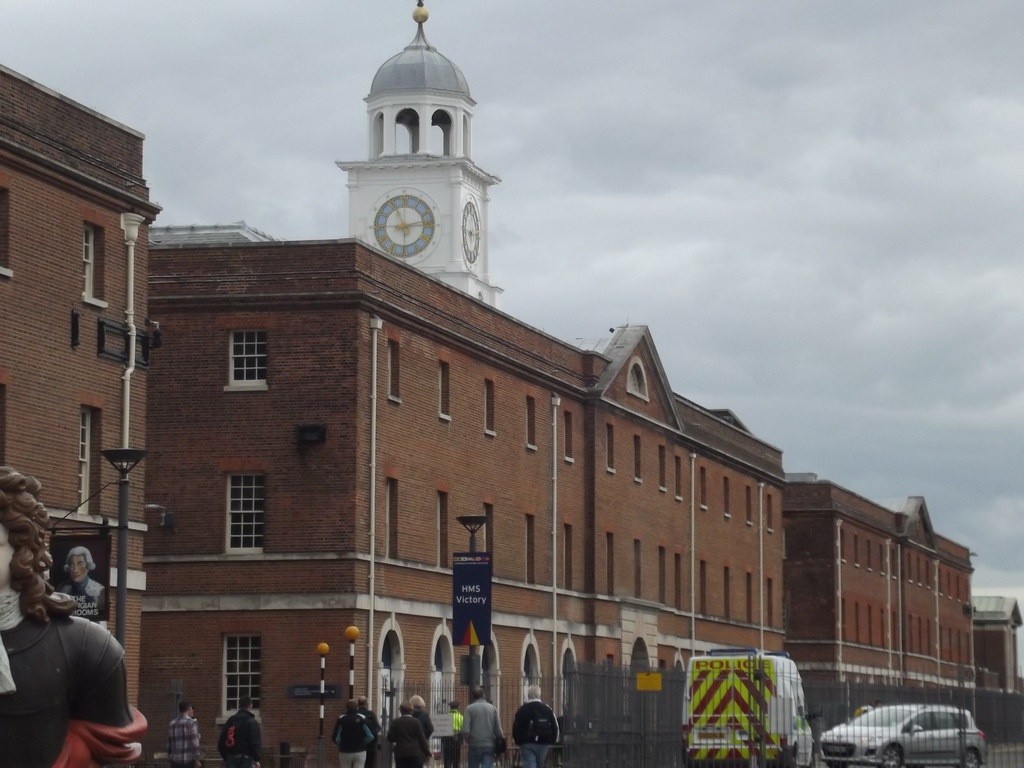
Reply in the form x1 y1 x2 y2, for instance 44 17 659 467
144 500 177 530
145 317 166 348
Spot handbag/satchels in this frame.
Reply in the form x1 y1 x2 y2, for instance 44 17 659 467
495 736 506 754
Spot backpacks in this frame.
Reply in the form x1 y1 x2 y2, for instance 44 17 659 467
519 704 558 745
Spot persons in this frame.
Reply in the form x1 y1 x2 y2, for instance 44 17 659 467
463 686 505 768
357 696 382 768
332 699 377 768
441 701 464 768
0 466 150 768
189 705 201 738
409 696 434 768
166 701 202 768
218 695 261 768
387 702 432 768
512 684 558 768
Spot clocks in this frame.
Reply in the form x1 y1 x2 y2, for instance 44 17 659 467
461 200 483 272
369 185 444 266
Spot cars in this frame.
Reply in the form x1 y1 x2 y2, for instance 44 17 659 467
819 704 988 768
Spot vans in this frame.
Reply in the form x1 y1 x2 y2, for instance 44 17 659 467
682 646 823 768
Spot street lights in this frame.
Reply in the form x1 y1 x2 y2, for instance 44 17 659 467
98 447 151 640
346 626 360 700
317 642 330 768
455 513 489 699
